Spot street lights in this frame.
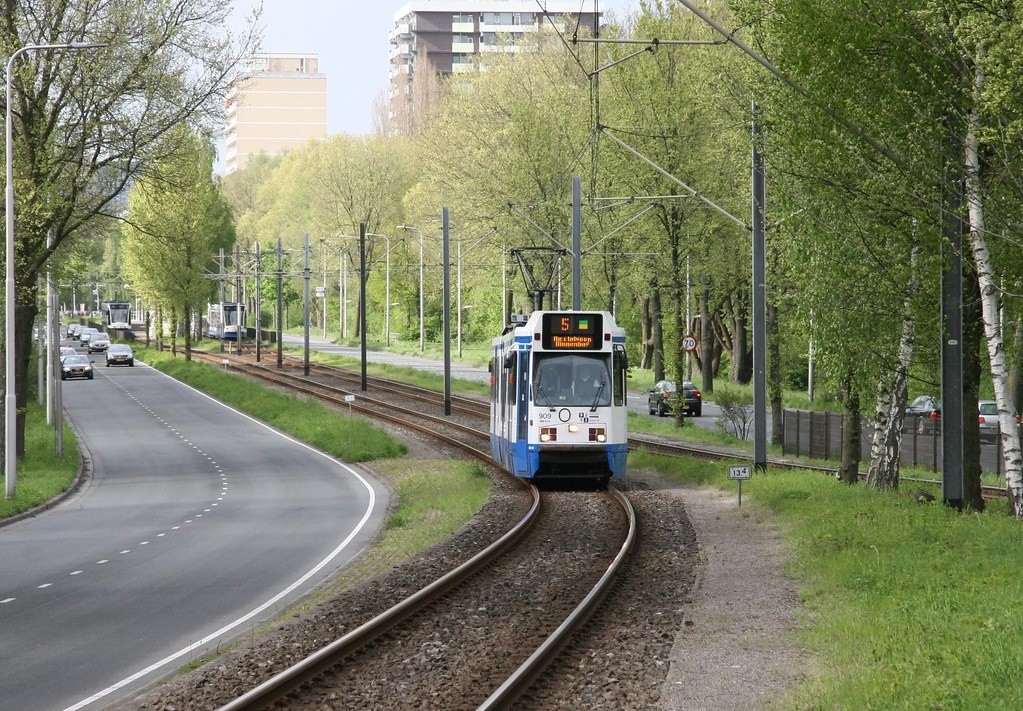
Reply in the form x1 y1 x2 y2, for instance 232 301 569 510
432 220 461 357
5 42 110 501
46 120 116 427
326 241 347 339
340 234 359 239
365 233 390 347
396 226 424 352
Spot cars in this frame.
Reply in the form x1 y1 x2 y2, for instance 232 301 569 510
80 328 99 347
60 347 77 366
89 311 99 317
87 333 113 355
105 344 136 367
66 324 81 338
60 355 96 381
647 380 702 417
979 400 1021 441
901 396 942 436
44 333 68 348
72 326 87 341
34 328 45 341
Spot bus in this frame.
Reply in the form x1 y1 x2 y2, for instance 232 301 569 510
488 310 629 490
208 302 248 340
101 301 132 330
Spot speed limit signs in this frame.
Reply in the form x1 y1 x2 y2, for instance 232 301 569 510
682 336 697 351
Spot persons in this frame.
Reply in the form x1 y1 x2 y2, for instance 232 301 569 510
578 367 599 387
